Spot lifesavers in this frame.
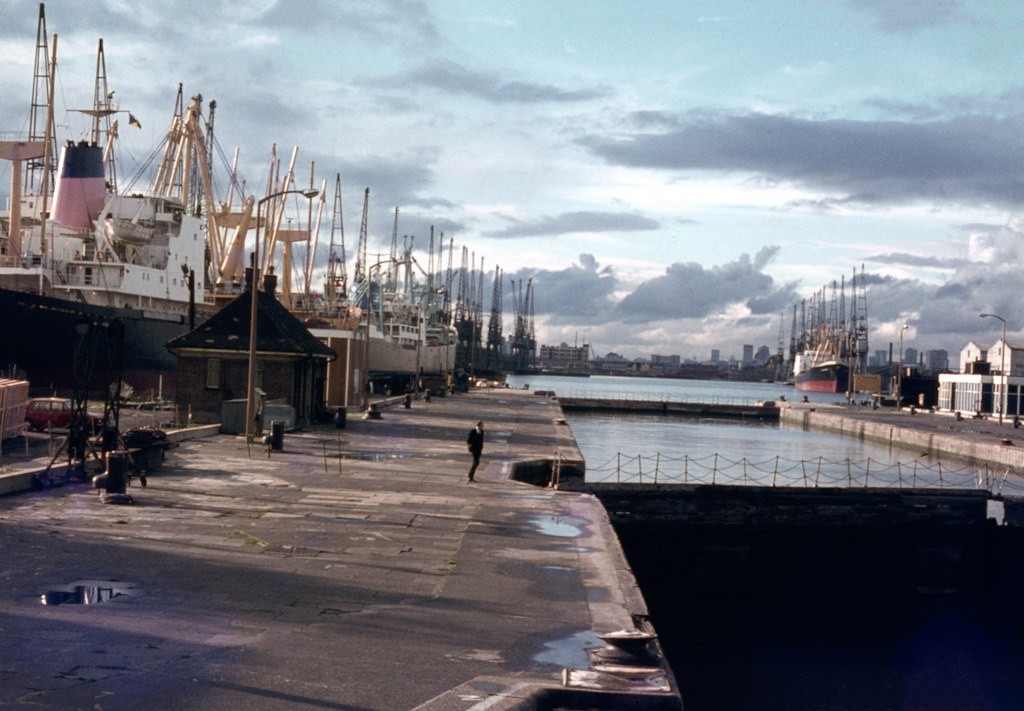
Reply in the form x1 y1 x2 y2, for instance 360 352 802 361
97 251 104 260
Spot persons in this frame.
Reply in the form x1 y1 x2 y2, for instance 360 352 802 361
466 419 484 483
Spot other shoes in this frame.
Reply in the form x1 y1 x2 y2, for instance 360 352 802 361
465 473 474 483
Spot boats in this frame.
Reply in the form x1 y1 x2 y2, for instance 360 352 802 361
1 0 538 402
792 337 853 397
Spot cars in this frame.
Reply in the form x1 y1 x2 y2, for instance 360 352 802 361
26 397 107 434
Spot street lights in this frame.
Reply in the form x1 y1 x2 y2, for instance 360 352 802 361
243 186 320 439
896 323 912 411
979 312 1007 425
